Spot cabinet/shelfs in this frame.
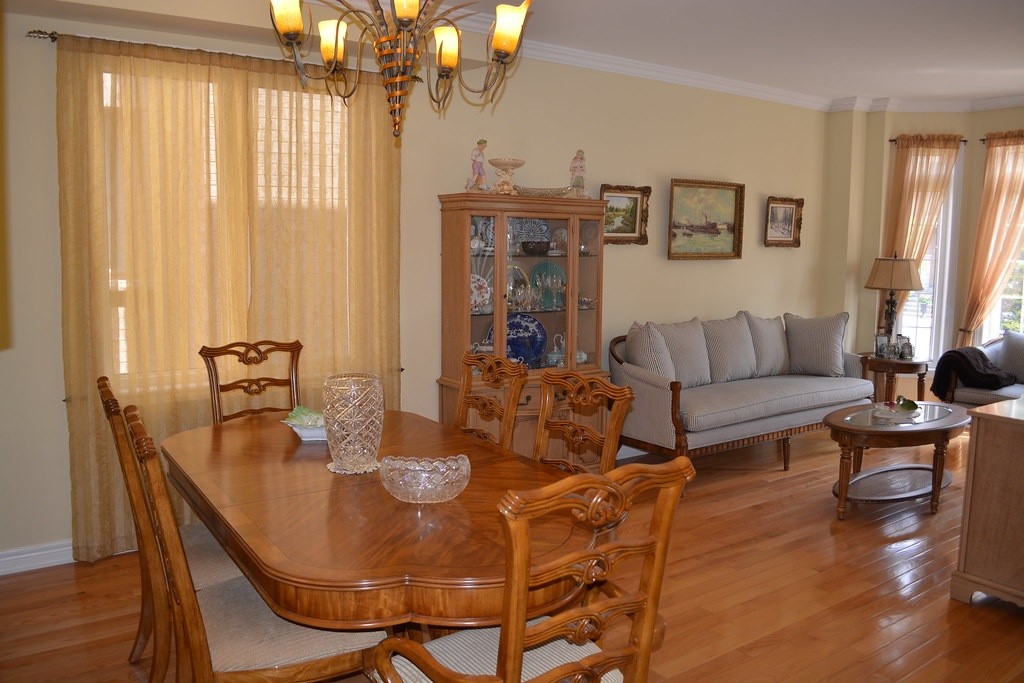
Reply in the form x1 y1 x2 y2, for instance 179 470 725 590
437 192 611 475
950 398 1024 607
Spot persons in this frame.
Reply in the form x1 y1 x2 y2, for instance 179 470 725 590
470 139 487 190
570 150 587 197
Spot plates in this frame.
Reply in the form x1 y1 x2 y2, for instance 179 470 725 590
874 410 920 419
468 212 570 309
875 400 917 415
486 313 547 365
513 185 573 198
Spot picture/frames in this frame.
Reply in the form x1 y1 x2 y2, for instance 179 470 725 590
902 343 914 359
882 344 899 359
599 184 652 246
897 336 910 354
668 178 746 261
875 335 890 354
764 196 804 248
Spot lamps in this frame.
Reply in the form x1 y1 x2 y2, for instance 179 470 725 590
864 251 925 343
269 0 534 138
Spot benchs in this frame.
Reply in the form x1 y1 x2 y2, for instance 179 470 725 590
608 335 875 499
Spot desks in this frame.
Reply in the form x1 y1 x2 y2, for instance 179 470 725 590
161 410 617 646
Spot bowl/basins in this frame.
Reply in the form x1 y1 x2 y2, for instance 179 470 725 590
381 454 471 504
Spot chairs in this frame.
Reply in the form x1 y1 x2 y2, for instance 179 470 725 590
944 337 1024 409
198 339 304 426
372 456 697 683
96 375 393 683
454 351 527 452
532 370 636 476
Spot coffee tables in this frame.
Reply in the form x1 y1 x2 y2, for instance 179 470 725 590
823 401 972 520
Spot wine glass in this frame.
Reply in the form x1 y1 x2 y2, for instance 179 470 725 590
514 285 527 311
545 273 562 309
534 272 548 309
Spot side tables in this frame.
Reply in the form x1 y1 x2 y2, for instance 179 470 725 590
859 353 929 403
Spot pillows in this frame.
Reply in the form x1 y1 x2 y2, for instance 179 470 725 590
784 312 849 377
746 311 791 378
701 311 757 383
651 316 711 388
624 321 676 381
997 329 1024 384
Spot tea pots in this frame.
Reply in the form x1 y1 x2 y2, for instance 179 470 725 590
548 329 587 366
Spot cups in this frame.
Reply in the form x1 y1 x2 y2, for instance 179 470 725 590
324 374 384 471
